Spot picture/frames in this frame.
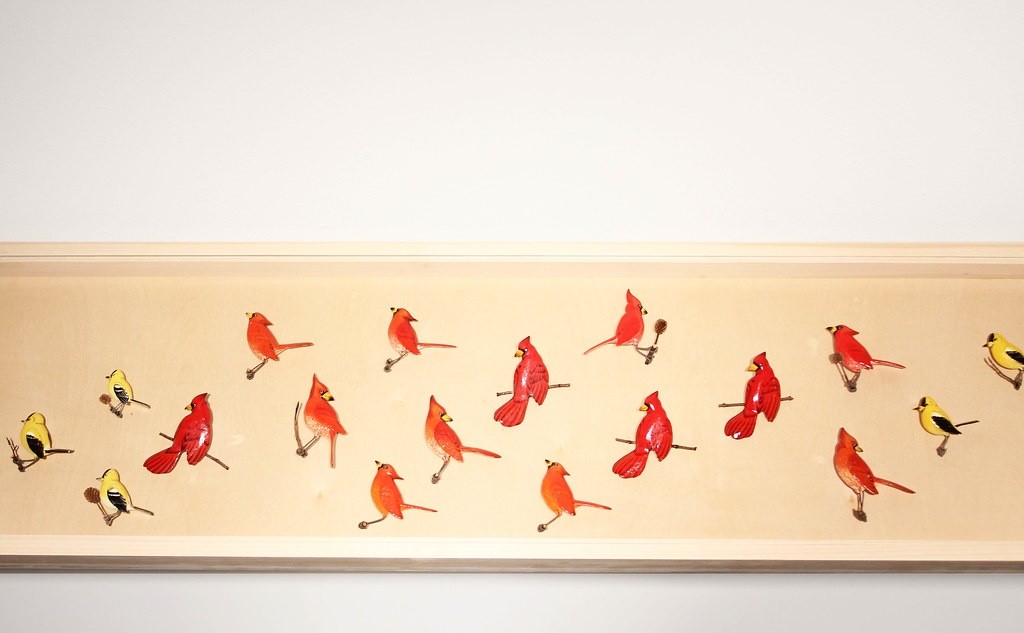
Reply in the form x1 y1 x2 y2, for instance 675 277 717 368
0 240 1023 575
